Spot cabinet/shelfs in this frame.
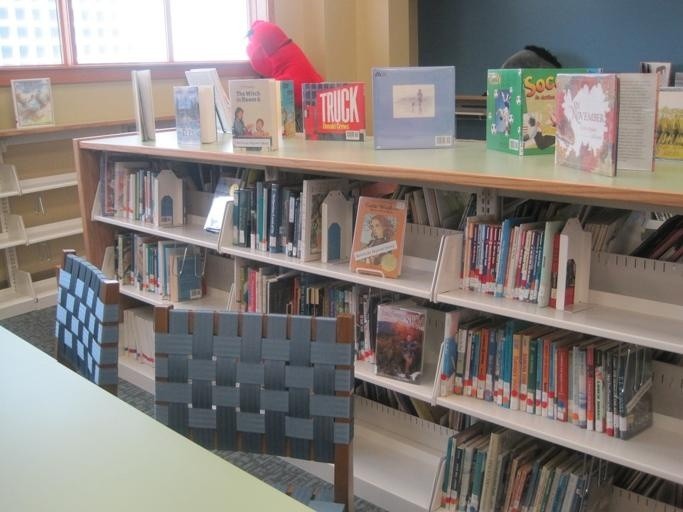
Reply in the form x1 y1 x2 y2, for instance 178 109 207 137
71 128 683 512
0 116 177 320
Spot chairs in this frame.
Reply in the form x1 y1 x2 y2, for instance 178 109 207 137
55 248 119 397
155 302 355 512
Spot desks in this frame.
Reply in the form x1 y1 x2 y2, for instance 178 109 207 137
0 323 318 512
